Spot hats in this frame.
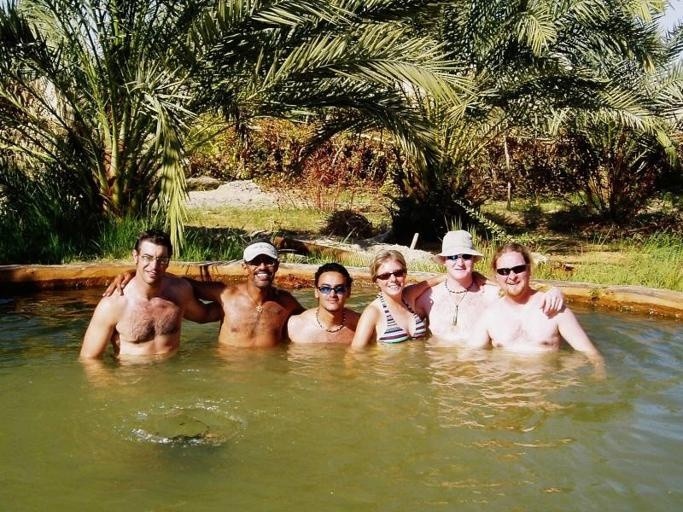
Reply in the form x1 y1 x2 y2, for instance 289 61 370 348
431 230 484 264
244 241 279 263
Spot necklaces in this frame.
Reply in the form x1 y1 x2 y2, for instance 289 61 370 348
444 277 476 294
246 284 274 313
444 277 476 326
314 304 346 333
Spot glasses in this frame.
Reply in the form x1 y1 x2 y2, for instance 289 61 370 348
137 253 170 264
317 285 349 294
495 265 528 276
445 255 474 260
376 269 405 280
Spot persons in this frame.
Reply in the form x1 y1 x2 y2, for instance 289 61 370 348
102 242 308 348
283 263 376 344
351 250 448 343
79 230 221 364
415 230 564 347
458 242 600 359
